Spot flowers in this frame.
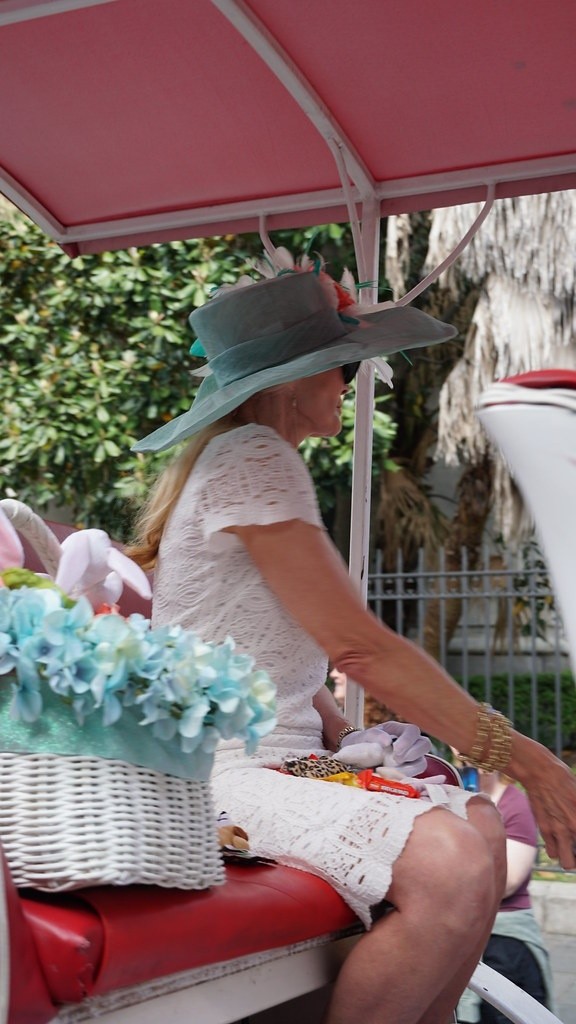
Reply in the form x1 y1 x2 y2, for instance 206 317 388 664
1 586 279 758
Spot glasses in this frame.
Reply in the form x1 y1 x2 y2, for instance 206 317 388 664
339 361 362 384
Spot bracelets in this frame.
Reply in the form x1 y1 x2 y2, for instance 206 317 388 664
473 703 513 773
335 726 359 753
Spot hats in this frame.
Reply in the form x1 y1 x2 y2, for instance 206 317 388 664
129 270 460 453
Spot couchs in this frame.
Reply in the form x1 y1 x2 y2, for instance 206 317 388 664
0 519 464 1024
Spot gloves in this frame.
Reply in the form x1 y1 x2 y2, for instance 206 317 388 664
340 718 432 778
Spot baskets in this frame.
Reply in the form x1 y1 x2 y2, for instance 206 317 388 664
4 749 226 895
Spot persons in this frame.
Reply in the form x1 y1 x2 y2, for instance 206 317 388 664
125 272 575 1024
